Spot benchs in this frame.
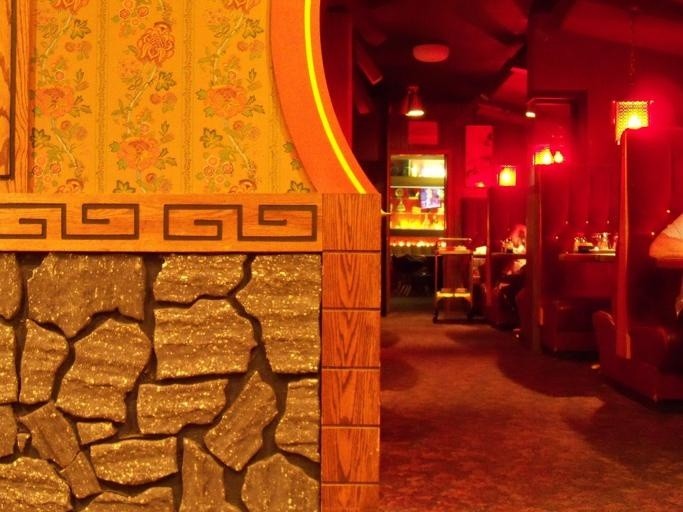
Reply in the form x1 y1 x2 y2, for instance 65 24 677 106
471 235 683 402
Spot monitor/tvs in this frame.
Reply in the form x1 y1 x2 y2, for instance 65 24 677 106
420 188 441 210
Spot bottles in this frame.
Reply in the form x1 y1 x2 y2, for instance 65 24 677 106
574 236 585 250
600 232 609 250
506 237 513 253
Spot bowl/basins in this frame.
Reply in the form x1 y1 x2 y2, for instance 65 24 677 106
578 244 593 252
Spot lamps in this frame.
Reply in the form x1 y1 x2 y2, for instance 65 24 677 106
399 82 425 118
606 96 656 145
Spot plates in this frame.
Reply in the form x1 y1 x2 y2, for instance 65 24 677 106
589 250 616 254
